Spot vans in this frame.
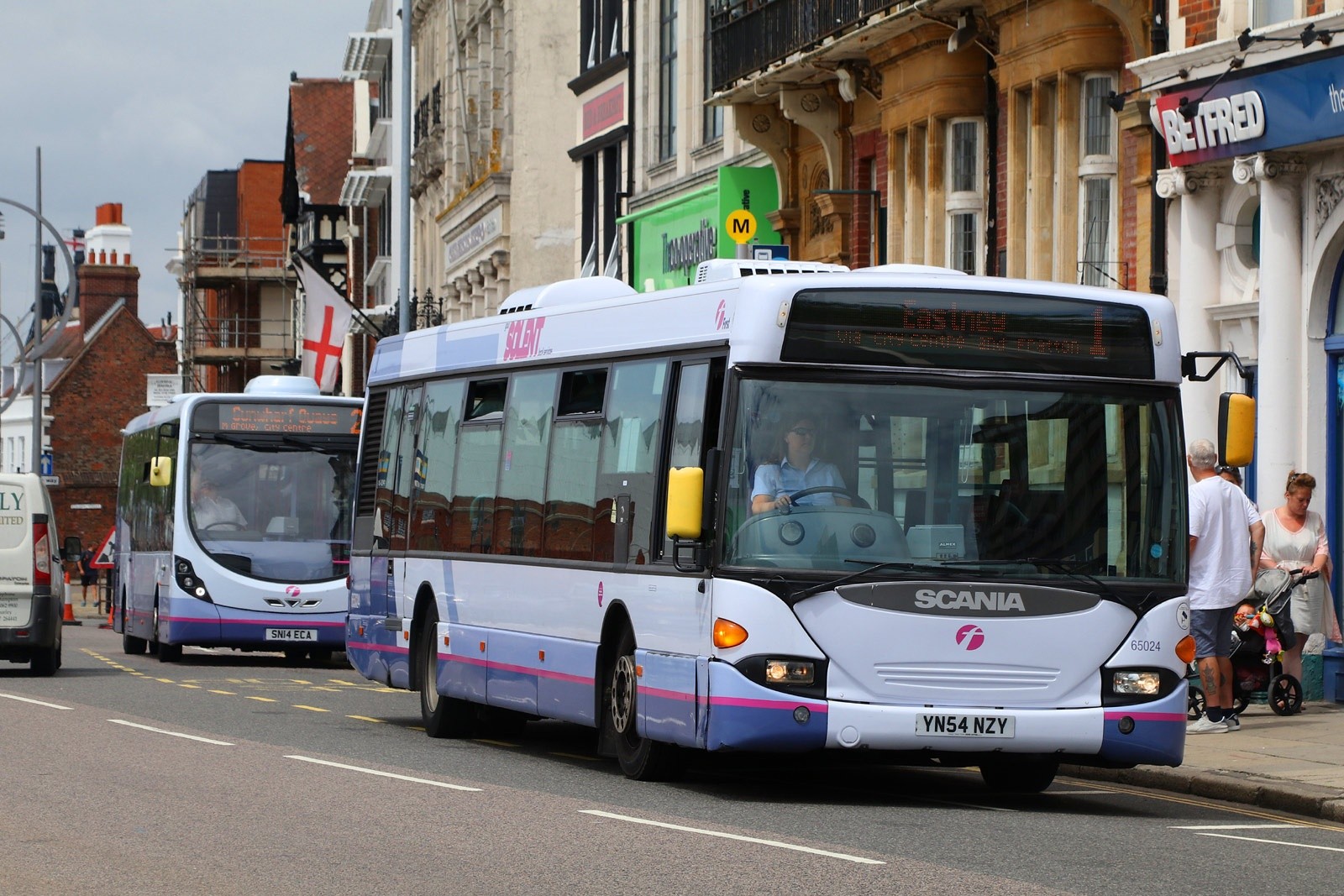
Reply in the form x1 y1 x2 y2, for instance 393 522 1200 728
0 474 83 677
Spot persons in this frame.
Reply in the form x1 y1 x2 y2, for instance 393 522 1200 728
749 415 853 513
62 540 100 608
1186 440 1343 734
194 471 249 531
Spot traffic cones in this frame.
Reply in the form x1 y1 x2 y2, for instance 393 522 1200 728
62 571 82 624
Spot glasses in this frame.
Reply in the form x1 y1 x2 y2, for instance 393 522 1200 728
1214 464 1236 475
1285 473 1299 491
788 427 819 436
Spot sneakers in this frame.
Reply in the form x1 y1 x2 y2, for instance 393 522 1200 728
1225 713 1241 730
1186 711 1228 734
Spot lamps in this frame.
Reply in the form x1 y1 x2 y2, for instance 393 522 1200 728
1105 68 1189 113
1178 58 1245 121
837 60 864 102
1238 23 1344 52
944 9 978 52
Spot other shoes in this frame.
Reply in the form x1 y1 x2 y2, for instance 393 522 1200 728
93 601 99 607
81 602 86 606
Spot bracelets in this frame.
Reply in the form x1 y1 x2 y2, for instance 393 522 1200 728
1313 565 1321 574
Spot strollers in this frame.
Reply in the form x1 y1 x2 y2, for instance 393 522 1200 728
1185 566 1320 721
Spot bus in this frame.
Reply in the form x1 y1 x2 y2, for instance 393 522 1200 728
114 374 366 668
346 259 1257 798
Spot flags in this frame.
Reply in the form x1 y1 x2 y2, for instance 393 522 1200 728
300 259 352 394
